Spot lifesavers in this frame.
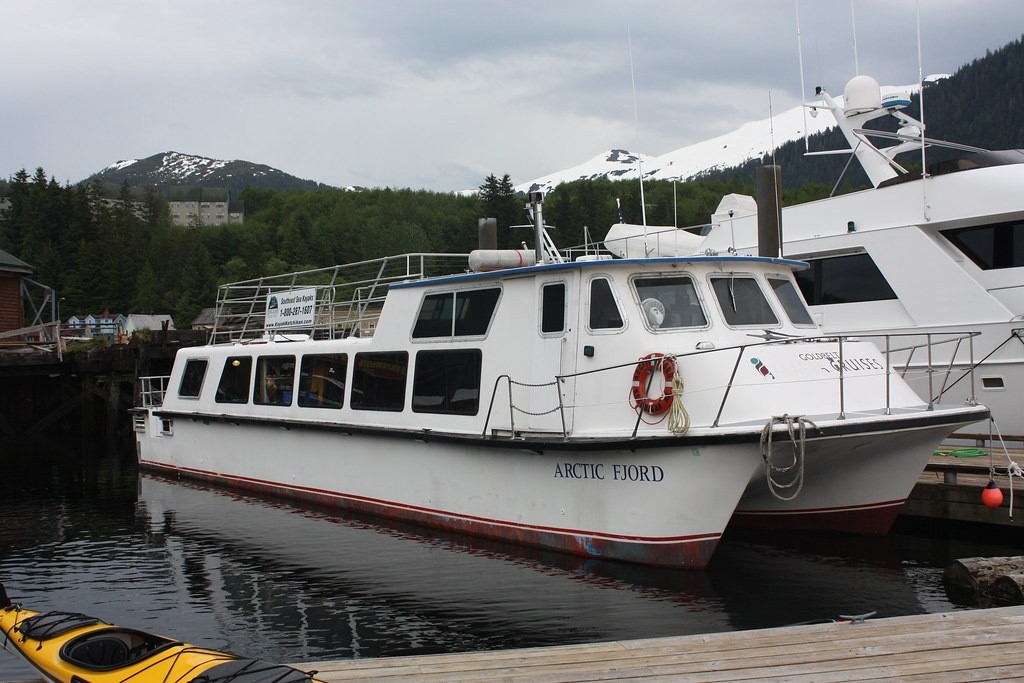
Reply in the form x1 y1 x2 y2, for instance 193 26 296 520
631 351 678 417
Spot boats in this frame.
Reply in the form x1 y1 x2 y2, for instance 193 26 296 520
0 583 327 683
603 1 1024 450
125 192 991 572
